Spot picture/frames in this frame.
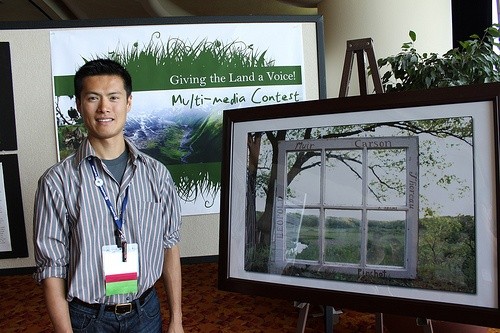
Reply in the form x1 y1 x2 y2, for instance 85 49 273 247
218 81 499 329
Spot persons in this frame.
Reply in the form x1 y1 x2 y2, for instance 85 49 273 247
31 59 184 333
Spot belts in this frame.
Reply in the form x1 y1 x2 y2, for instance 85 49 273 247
69 285 154 315
